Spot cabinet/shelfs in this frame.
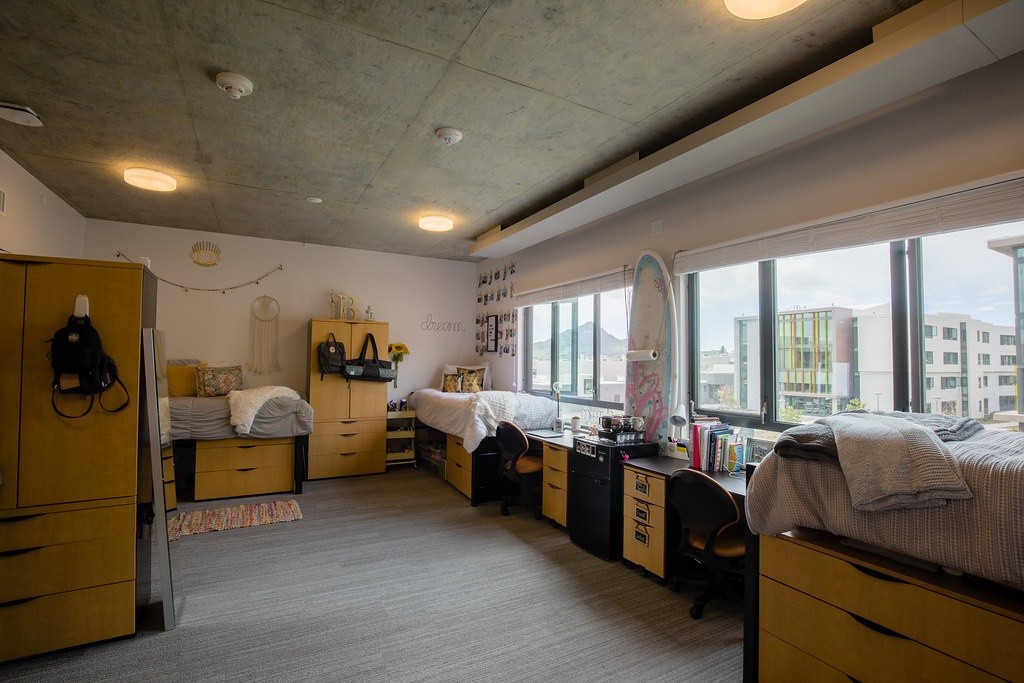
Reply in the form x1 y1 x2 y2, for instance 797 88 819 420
540 442 571 531
623 463 665 586
0 254 143 663
173 439 303 496
415 416 497 507
308 320 389 481
384 408 417 470
741 523 1024 683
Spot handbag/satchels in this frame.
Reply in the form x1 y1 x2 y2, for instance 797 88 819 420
343 332 396 382
317 332 346 381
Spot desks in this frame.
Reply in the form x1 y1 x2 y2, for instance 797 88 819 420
625 455 751 499
523 426 595 451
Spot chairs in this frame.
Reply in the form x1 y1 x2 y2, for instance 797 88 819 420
494 421 543 516
669 467 747 619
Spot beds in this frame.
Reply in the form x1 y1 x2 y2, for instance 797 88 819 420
409 389 564 454
168 385 314 439
744 411 1024 586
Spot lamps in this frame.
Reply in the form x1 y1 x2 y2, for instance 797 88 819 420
124 167 178 193
670 404 687 440
418 216 453 233
553 382 563 417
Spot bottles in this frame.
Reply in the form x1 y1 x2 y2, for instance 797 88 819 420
400 399 407 411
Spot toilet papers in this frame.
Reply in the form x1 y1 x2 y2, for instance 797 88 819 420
626 349 659 362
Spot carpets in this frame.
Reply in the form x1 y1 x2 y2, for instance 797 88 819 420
168 499 303 542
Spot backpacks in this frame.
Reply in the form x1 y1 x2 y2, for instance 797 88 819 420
44 312 130 419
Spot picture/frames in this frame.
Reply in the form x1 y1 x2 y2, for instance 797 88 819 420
746 437 775 463
487 315 499 352
554 417 564 432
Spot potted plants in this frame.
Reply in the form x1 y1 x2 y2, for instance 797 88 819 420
571 415 581 431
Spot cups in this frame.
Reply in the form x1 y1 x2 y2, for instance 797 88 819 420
554 418 564 434
572 416 581 431
599 414 644 433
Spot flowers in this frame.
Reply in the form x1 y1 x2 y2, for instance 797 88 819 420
387 342 410 388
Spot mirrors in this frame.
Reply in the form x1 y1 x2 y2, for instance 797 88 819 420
142 328 186 629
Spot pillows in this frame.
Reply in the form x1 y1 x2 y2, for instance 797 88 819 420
442 374 463 394
458 368 486 393
168 362 207 396
440 362 493 391
196 364 243 396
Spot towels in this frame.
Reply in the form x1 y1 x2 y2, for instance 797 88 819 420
773 409 985 514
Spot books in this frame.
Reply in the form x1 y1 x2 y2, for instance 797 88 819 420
689 422 743 472
